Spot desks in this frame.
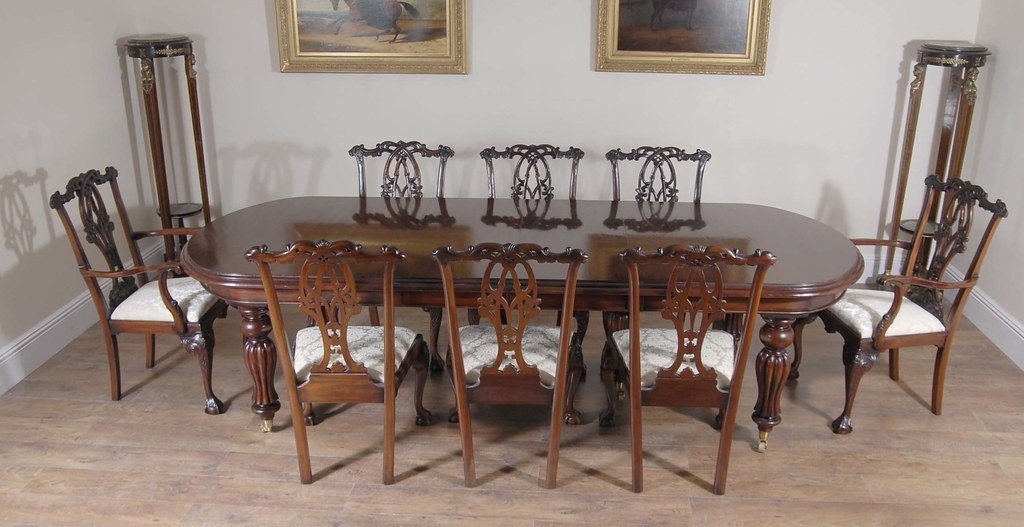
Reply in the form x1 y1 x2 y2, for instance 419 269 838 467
177 194 864 460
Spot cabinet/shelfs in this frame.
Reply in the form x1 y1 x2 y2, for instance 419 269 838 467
877 39 993 304
125 35 212 262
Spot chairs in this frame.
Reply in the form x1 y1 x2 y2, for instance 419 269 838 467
603 199 707 233
786 174 1008 436
48 167 231 415
477 144 585 384
480 198 582 231
597 241 778 497
353 197 457 230
348 140 454 385
599 146 712 384
432 241 589 490
246 238 434 490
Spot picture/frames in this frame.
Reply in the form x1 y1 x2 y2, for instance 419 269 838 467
595 0 772 76
275 0 468 76
